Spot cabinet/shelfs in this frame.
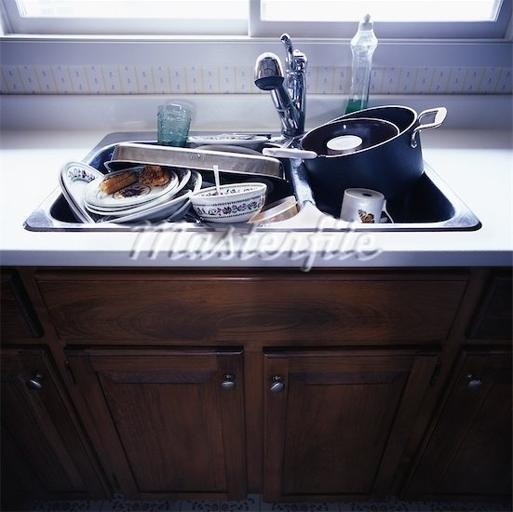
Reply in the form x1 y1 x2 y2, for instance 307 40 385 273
0 267 513 503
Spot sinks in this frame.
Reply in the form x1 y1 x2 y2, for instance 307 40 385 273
24 130 300 233
301 130 482 232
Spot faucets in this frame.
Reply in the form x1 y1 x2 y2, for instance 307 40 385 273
253 34 306 137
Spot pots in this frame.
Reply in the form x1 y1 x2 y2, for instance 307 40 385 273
262 104 447 202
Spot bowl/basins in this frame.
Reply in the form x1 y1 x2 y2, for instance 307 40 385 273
189 182 266 224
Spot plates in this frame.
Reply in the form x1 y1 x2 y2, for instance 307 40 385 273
57 161 202 225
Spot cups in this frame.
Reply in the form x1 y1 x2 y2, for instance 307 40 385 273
157 104 193 146
338 187 395 225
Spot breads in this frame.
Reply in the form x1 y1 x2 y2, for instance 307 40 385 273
101 171 137 194
